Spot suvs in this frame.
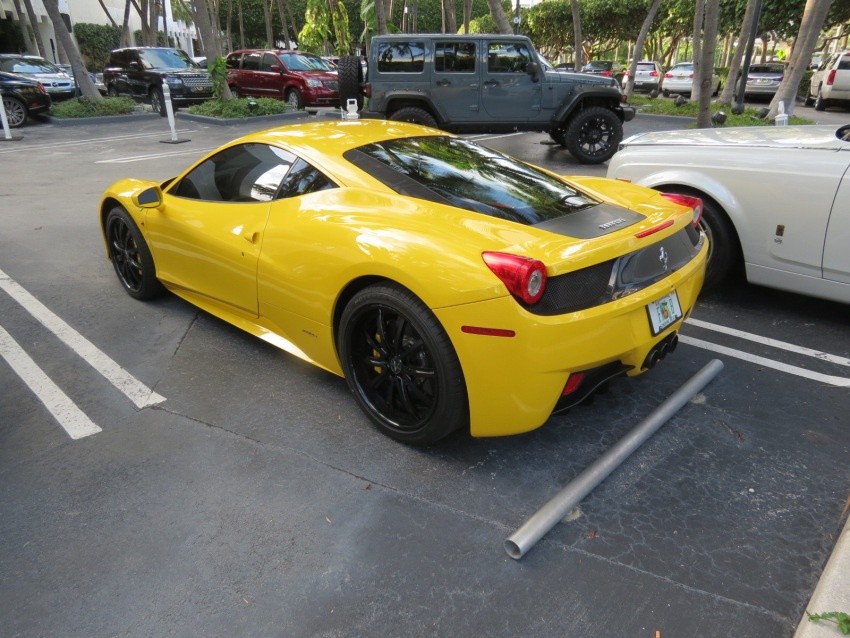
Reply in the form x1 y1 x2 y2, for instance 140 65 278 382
103 46 219 117
336 32 636 164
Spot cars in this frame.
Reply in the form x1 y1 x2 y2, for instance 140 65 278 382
0 52 104 129
554 60 722 99
606 122 850 308
734 63 785 101
192 48 370 112
805 49 850 111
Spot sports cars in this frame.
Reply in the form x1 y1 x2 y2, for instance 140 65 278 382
100 118 711 451
